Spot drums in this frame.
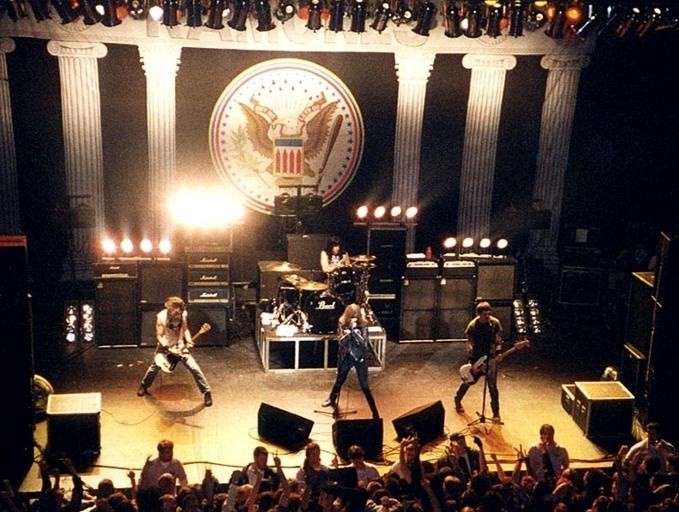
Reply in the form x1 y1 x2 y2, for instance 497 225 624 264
304 291 345 333
329 266 358 294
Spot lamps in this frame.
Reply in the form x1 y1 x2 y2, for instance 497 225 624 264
8 0 28 23
256 0 277 32
205 0 226 30
510 1 525 37
187 0 204 26
638 8 662 37
53 0 79 23
329 0 347 31
29 0 52 21
306 0 324 31
598 4 625 41
463 0 484 38
487 3 506 36
228 1 252 30
615 6 643 40
100 1 123 27
77 1 106 26
545 1 567 40
162 0 182 25
445 1 466 38
413 0 438 35
351 0 368 33
572 5 604 42
370 0 392 31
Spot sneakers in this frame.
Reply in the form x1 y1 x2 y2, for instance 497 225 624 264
322 398 331 406
493 414 501 421
455 397 465 412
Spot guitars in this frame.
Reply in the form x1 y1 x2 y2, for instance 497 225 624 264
459 340 530 386
154 322 211 374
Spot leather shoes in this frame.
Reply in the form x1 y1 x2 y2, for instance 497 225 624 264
137 385 145 396
204 393 212 406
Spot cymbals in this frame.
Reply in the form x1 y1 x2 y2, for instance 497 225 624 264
348 254 377 261
263 260 302 272
295 281 329 290
282 273 308 285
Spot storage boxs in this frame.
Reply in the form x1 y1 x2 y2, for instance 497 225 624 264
574 380 634 440
46 391 102 458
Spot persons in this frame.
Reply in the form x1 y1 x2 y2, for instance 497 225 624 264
272 424 679 512
2 460 140 512
320 236 351 273
137 297 213 407
139 441 275 512
322 304 380 419
455 301 504 426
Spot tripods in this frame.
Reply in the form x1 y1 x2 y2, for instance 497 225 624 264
467 327 504 426
313 330 357 420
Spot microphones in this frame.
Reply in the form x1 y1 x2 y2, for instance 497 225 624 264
486 316 493 328
